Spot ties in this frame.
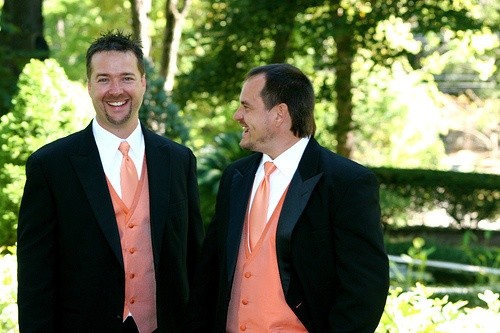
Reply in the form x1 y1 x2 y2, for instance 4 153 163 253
249 161 277 253
118 141 140 211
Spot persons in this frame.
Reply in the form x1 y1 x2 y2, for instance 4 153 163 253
15 28 210 333
187 63 392 333
